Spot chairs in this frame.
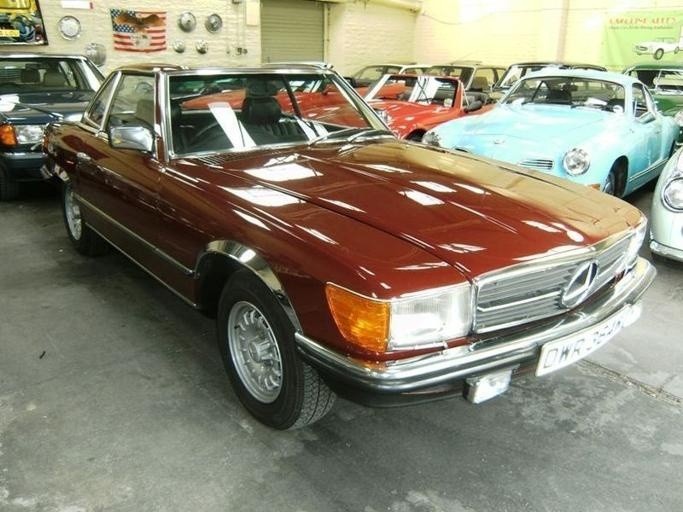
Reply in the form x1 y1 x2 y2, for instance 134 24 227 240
134 96 309 155
544 89 572 105
604 98 624 114
19 68 71 91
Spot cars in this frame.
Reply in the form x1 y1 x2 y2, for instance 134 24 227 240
649 150 683 263
180 57 683 199
1 53 134 203
37 59 659 432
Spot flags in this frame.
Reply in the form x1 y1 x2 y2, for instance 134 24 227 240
109 7 166 54
